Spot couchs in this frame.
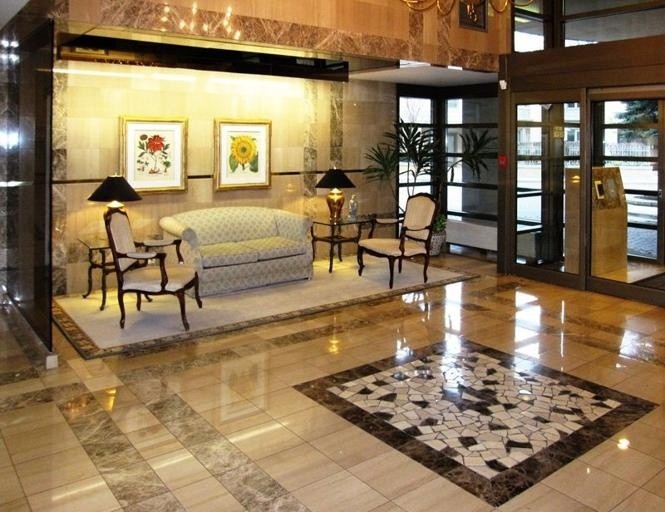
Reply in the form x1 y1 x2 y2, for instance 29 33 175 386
157 208 313 298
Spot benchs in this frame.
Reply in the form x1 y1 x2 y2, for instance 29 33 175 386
443 214 542 264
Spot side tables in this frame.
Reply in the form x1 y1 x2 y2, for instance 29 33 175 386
309 215 368 273
77 235 114 312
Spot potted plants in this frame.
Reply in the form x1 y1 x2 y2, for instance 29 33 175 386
364 123 496 256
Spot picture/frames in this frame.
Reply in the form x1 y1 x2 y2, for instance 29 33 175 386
214 117 273 193
118 115 189 196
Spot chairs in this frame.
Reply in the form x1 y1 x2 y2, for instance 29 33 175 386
355 193 439 290
104 204 203 333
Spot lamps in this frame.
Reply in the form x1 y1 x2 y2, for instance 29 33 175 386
315 167 355 224
85 174 142 220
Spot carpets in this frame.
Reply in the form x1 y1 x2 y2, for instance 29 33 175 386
50 253 479 362
632 269 665 290
